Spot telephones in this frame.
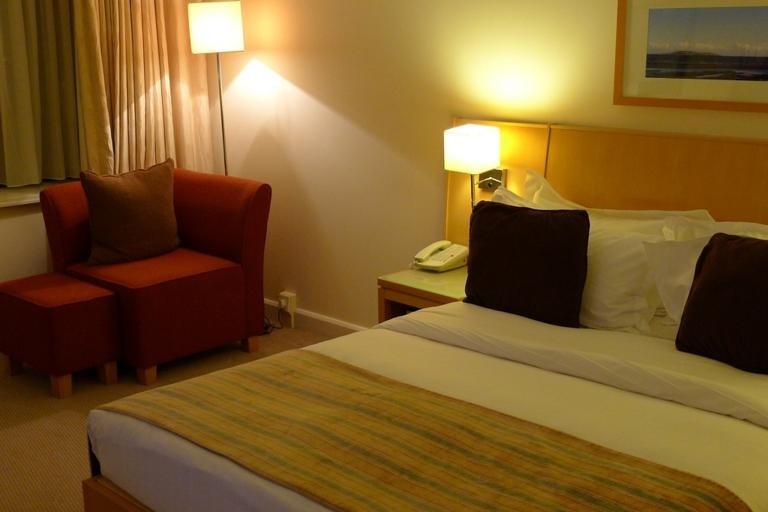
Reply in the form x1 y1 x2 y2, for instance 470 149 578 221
413 239 470 273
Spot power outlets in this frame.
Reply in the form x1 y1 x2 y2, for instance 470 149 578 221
279 292 295 313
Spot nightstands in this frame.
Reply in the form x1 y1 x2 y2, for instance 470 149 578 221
377 261 467 321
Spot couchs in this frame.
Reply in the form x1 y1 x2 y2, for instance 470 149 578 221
1 273 116 398
38 159 271 385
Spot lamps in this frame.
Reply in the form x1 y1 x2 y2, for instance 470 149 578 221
441 123 502 211
186 1 246 177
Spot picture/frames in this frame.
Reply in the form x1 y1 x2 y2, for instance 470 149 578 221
613 1 767 112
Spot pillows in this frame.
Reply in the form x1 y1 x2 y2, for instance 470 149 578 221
463 202 590 329
644 217 768 330
83 158 180 265
676 232 768 375
520 171 716 341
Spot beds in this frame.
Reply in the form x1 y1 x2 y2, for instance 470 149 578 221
79 114 768 511
